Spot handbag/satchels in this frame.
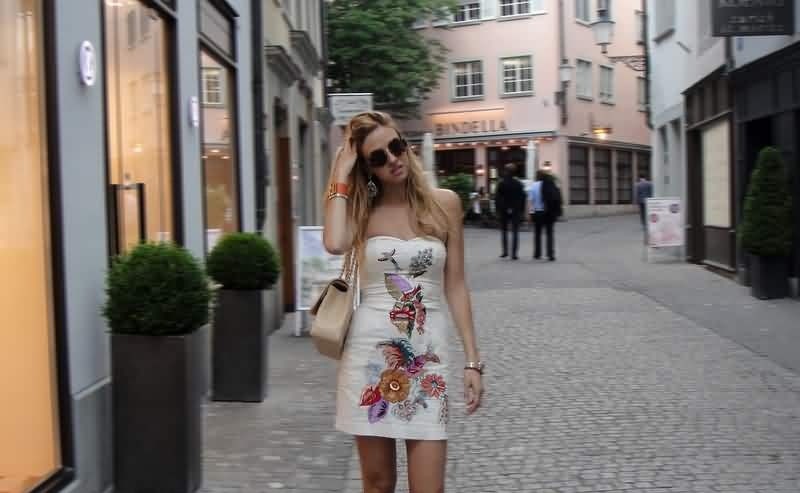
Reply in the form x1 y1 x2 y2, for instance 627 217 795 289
309 276 360 362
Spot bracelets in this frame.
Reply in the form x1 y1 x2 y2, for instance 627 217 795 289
327 182 350 198
328 193 348 200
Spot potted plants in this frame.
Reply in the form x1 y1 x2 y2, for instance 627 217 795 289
736 146 797 299
103 239 217 490
201 228 281 405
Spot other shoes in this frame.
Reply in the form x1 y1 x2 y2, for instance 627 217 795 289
500 254 510 259
548 256 557 261
511 257 519 260
534 256 540 260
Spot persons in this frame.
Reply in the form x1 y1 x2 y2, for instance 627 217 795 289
494 163 526 260
538 159 562 190
635 173 654 231
321 110 488 493
527 169 563 261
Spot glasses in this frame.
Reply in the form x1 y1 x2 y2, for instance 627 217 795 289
368 139 409 170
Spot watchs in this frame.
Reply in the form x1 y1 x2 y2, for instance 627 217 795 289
463 360 487 374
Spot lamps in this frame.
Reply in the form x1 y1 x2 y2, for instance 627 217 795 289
558 56 574 86
590 8 619 52
